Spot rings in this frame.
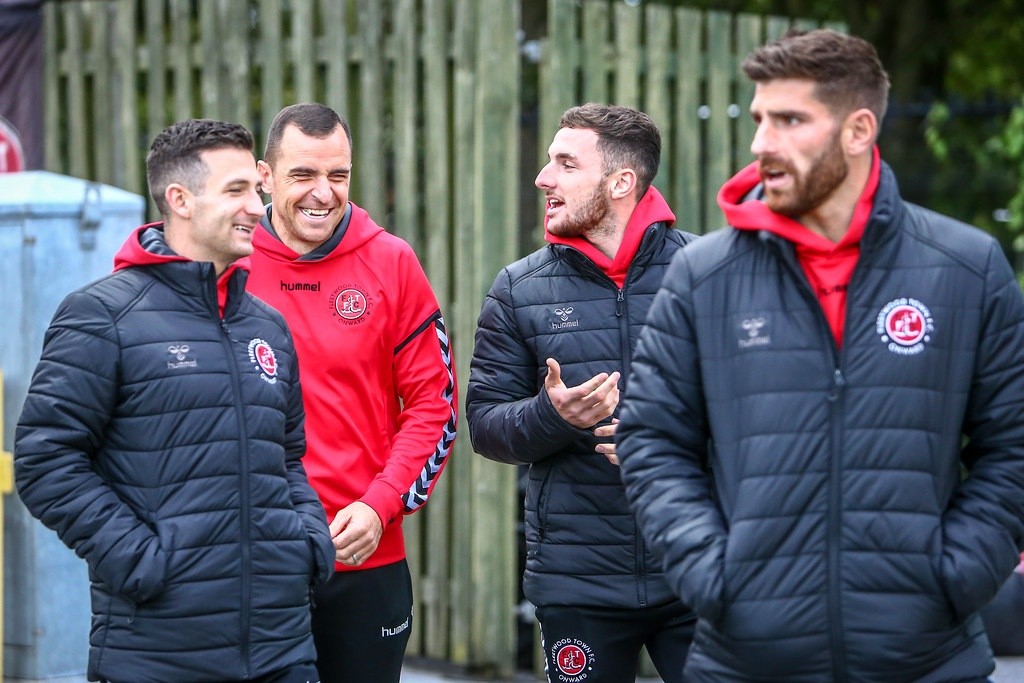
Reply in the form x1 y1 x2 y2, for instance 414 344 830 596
353 554 357 563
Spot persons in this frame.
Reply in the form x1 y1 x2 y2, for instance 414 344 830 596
614 29 1024 683
465 104 700 683
235 102 460 683
13 119 336 683
